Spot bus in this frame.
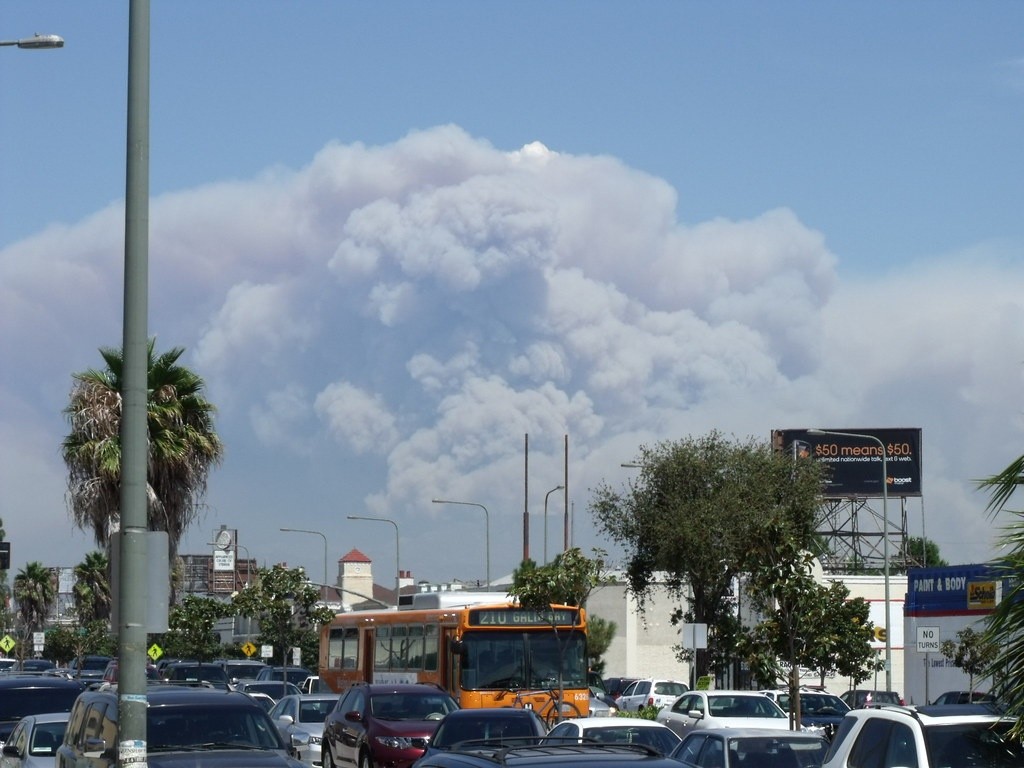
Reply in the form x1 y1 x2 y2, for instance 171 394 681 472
316 601 591 728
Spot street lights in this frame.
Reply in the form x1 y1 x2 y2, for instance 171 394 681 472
280 528 328 609
808 427 893 694
347 516 401 609
544 484 565 570
433 497 491 593
206 542 251 662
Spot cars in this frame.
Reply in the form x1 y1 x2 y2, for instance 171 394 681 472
0 651 1024 768
